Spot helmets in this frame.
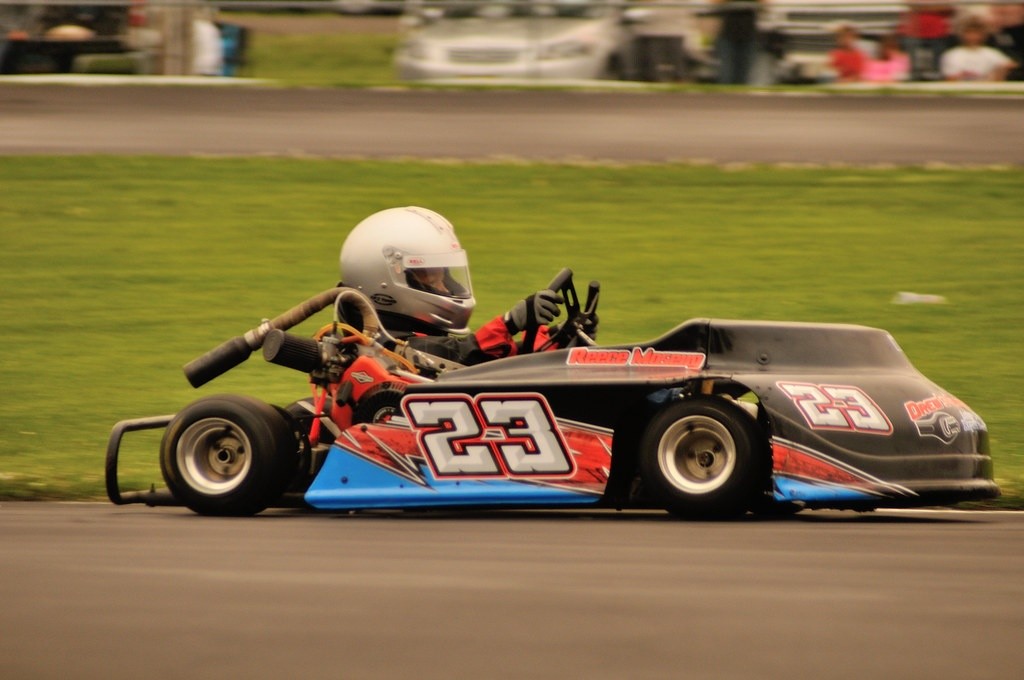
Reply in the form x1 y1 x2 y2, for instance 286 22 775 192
340 205 476 337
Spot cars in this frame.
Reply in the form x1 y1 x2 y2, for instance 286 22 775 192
393 0 1024 86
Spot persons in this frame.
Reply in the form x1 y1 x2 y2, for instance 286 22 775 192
339 206 597 379
617 0 1024 82
0 0 241 78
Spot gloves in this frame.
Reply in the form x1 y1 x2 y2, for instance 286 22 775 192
504 288 565 335
549 310 599 349
